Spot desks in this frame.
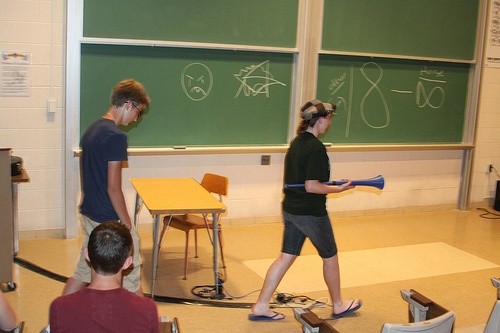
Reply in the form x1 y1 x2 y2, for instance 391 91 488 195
129 177 226 300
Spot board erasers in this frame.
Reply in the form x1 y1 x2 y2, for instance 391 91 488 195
173 146 185 149
323 143 331 146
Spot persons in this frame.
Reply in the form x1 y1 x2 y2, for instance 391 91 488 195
40 79 149 333
48 220 161 333
0 292 25 333
248 99 363 321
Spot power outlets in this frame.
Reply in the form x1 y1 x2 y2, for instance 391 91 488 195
485 163 493 175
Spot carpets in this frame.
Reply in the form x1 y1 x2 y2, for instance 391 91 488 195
240 240 500 293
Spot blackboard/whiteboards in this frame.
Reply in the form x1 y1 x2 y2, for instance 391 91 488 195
68 0 489 156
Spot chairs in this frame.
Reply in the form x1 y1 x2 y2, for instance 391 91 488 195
292 289 455 333
158 173 230 280
